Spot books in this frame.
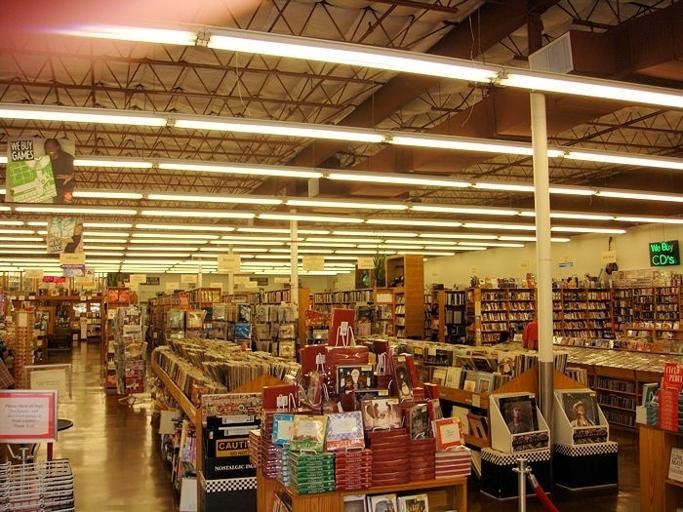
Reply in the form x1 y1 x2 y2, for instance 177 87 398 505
2 270 683 512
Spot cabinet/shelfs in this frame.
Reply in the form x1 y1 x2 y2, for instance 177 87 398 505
101 255 683 512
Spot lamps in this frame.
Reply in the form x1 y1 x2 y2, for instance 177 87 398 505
0 24 683 276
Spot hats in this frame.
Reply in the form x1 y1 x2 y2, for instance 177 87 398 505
573 400 583 416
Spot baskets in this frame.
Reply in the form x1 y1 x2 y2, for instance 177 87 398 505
0 459 75 512
